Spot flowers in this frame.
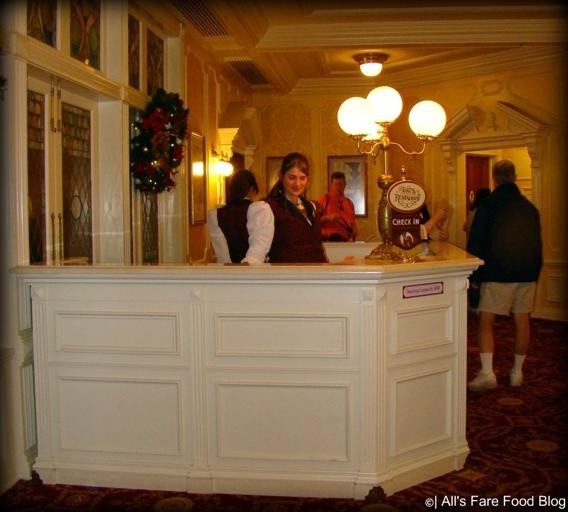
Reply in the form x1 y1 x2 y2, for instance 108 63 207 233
129 87 191 196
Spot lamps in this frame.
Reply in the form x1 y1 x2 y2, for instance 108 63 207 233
336 86 447 260
352 51 390 77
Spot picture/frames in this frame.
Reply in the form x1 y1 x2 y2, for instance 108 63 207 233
188 131 207 226
327 154 368 218
266 155 307 203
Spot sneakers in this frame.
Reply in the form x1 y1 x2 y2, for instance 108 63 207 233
468 373 497 390
509 371 524 387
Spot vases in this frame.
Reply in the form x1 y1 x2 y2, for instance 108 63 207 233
136 189 159 268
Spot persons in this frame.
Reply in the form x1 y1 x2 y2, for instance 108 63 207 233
317 171 358 242
463 187 493 320
241 152 329 264
467 160 543 393
420 202 450 242
207 170 260 263
